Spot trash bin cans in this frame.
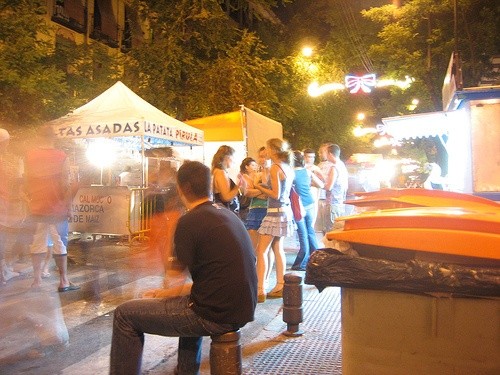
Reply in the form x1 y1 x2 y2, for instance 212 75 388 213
324 189 500 375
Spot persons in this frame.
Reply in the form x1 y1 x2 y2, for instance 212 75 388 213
110 161 259 375
213 139 348 303
0 126 82 292
399 146 443 190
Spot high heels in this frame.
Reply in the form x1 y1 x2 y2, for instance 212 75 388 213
267 283 285 297
257 288 266 303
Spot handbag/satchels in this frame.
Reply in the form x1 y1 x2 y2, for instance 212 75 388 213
290 186 306 221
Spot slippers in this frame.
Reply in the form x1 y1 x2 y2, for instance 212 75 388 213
58 282 80 292
31 283 40 292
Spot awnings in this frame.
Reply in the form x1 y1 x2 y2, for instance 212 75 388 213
370 109 462 146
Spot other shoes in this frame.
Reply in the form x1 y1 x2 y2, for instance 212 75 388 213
291 265 306 271
41 272 51 278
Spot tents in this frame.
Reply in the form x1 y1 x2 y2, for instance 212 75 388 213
45 80 205 146
182 103 283 158
